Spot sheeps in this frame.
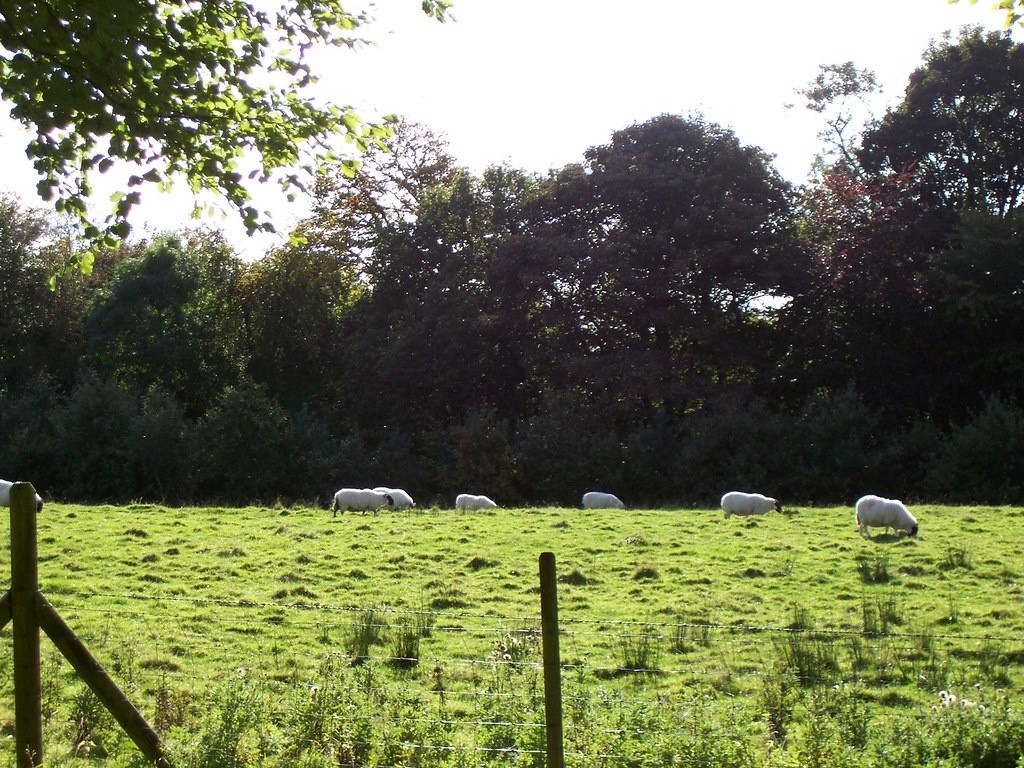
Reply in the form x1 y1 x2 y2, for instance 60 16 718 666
455 493 498 513
331 487 416 517
582 492 625 509
856 495 919 539
720 491 782 520
0 480 43 512
